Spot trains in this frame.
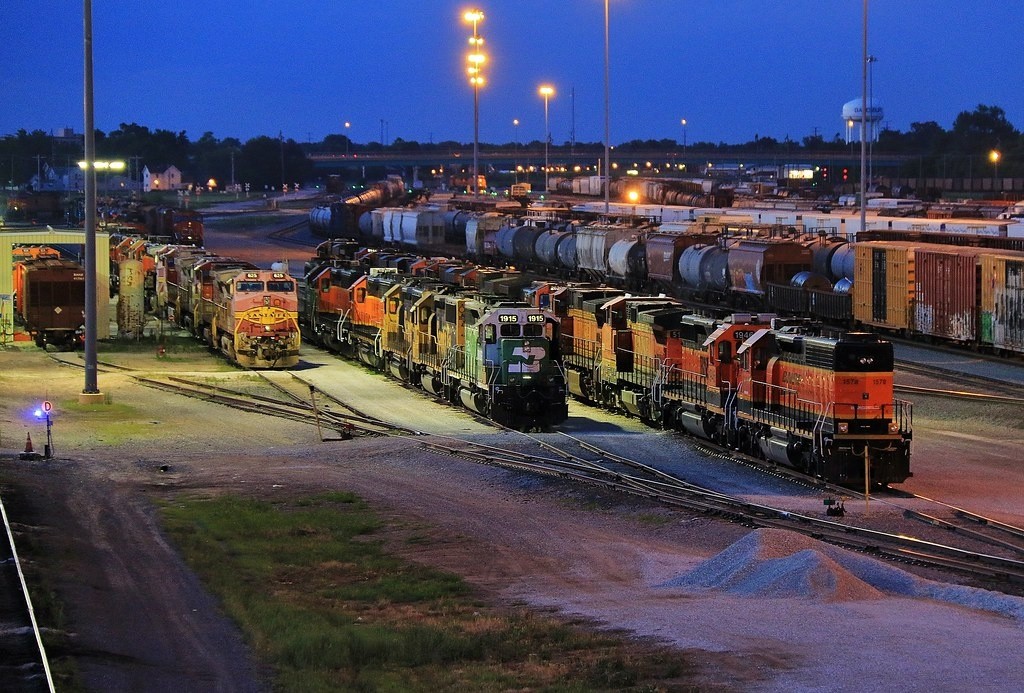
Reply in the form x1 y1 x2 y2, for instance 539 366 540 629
6 191 300 369
308 173 1024 357
301 239 912 492
12 246 86 348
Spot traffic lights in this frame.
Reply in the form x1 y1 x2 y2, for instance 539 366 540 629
843 169 848 180
824 168 828 177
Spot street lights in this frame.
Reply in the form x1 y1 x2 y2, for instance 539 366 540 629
346 122 350 158
991 150 998 180
538 85 557 190
513 118 519 160
682 120 687 152
848 120 854 159
461 8 489 198
